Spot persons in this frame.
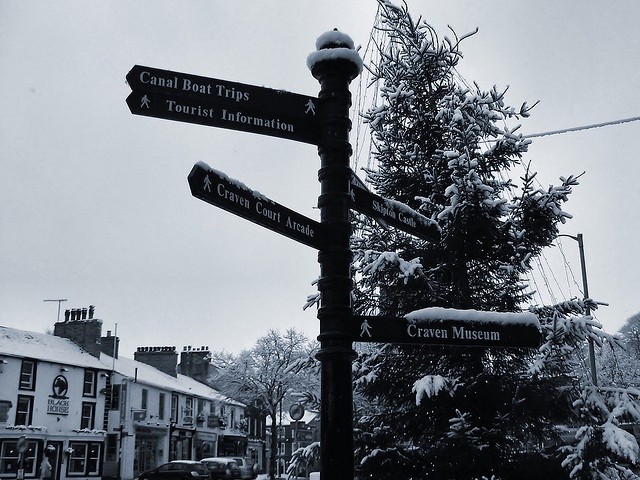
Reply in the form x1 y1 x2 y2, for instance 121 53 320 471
37 457 51 480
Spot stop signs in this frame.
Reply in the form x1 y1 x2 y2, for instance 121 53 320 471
289 404 304 421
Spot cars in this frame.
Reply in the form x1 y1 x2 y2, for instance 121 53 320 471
134 460 211 480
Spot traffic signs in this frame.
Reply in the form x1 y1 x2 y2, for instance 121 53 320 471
351 315 541 348
126 65 320 145
349 182 441 245
188 164 320 250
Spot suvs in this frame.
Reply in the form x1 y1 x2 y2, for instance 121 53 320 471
234 456 260 479
200 458 240 480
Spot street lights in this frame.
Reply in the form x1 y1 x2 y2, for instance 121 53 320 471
523 234 597 392
